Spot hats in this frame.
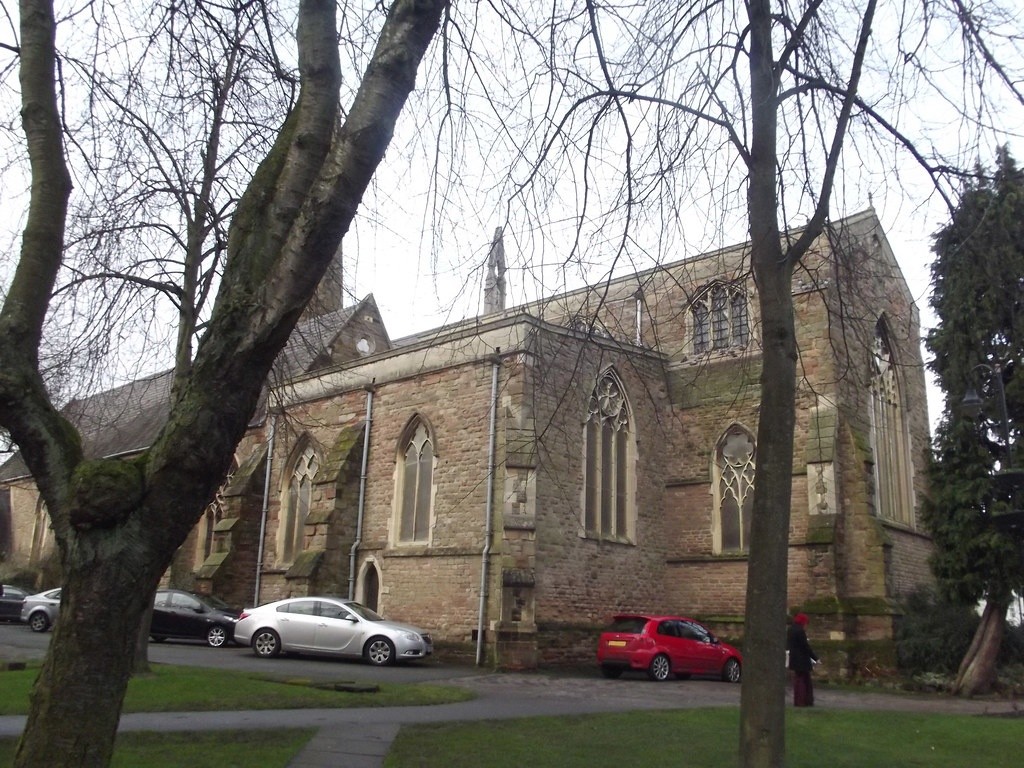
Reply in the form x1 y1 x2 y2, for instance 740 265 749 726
794 612 810 629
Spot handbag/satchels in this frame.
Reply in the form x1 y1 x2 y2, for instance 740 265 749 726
785 650 790 668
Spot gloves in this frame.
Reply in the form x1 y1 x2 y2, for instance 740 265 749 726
816 659 821 665
811 658 817 665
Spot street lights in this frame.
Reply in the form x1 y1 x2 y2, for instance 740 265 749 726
961 364 1010 466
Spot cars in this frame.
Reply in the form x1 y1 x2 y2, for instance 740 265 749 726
149 589 242 648
234 597 433 666
598 615 743 683
21 587 62 633
0 585 31 622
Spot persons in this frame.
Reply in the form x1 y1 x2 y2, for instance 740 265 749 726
786 613 822 707
